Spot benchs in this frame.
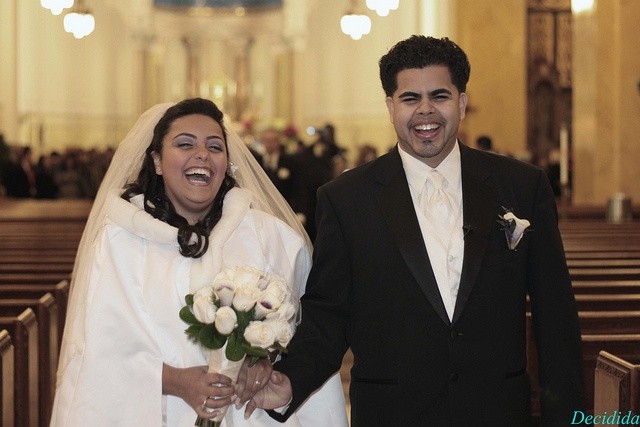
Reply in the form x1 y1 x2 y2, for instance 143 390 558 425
0 196 89 427
524 206 640 427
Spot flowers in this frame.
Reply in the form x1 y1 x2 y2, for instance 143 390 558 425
496 204 532 249
177 263 303 427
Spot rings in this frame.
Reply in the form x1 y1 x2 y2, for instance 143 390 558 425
256 381 260 385
203 398 209 406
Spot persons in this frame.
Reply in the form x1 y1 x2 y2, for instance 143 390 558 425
326 153 350 183
256 128 293 190
49 98 349 427
357 144 380 164
310 124 343 165
236 34 585 427
476 135 492 150
0 133 116 200
289 138 308 159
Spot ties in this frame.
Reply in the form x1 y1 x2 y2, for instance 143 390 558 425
423 171 457 252
